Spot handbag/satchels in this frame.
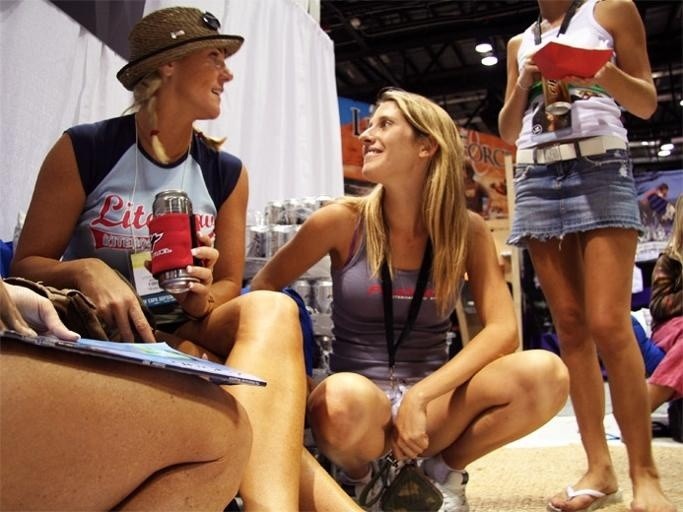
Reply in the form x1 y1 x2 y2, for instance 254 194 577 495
379 463 443 512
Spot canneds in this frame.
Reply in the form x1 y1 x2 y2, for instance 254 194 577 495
249 198 338 374
149 190 199 295
540 60 571 117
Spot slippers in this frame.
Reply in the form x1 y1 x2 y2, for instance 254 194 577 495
547 484 624 512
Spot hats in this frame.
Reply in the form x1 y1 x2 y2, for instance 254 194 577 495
115 5 246 93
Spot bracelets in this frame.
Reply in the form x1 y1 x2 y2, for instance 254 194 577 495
516 78 532 94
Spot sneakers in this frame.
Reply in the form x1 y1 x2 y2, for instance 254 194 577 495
417 453 470 512
338 462 400 512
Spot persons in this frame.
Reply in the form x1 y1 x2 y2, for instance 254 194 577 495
642 194 683 416
461 159 494 219
637 181 672 226
244 86 571 512
497 0 679 511
10 5 365 511
0 278 254 512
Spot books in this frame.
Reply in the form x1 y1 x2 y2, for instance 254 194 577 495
0 328 269 388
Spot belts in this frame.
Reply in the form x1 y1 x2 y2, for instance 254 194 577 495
514 136 628 165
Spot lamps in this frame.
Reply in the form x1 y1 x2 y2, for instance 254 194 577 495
474 33 500 67
657 139 673 157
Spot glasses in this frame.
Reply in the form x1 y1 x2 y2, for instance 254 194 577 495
170 10 222 38
